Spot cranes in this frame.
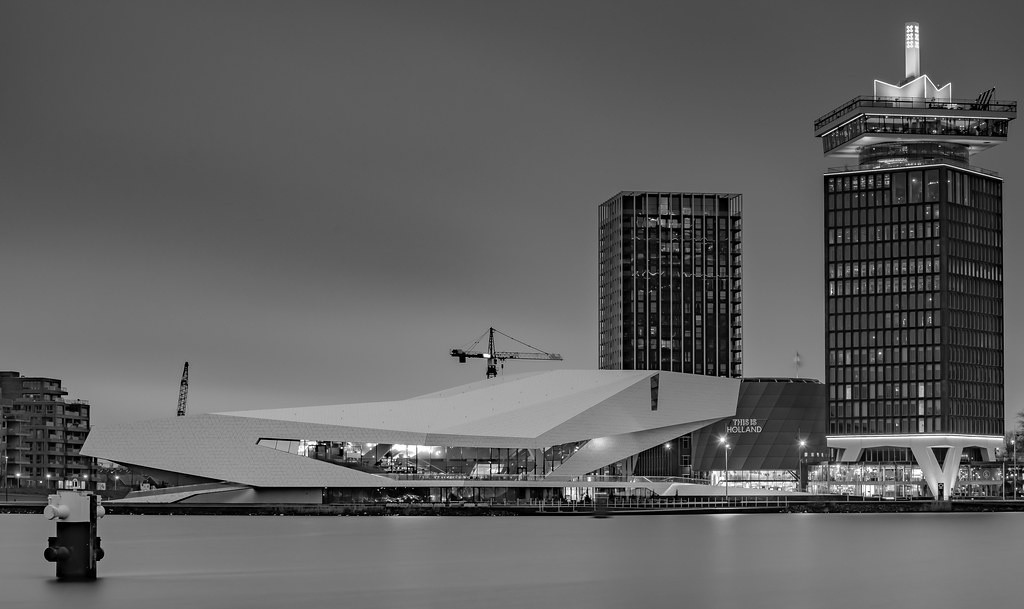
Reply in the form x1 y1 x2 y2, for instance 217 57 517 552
450 327 563 379
176 361 190 416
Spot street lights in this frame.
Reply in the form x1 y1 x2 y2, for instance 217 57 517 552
5 457 8 487
798 440 804 492
17 474 20 488
1010 439 1017 500
46 475 50 489
111 470 116 491
726 445 731 502
